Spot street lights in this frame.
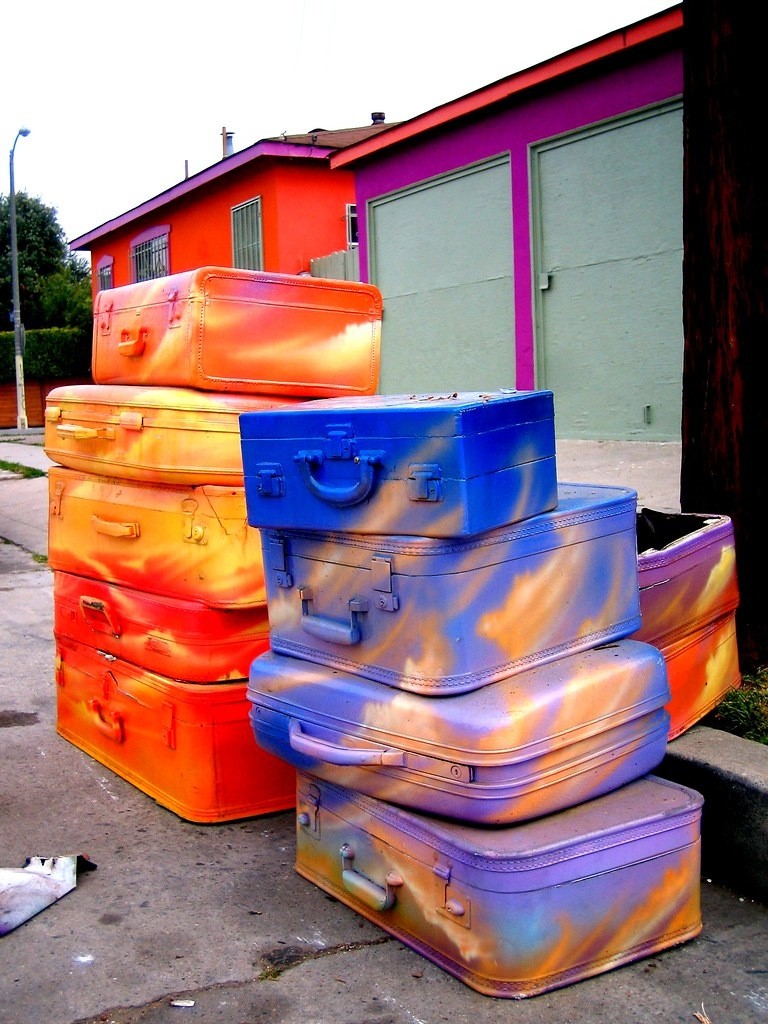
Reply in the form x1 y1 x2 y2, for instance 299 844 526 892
9 128 32 432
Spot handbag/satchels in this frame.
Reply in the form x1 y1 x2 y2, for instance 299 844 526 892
41 263 705 996
